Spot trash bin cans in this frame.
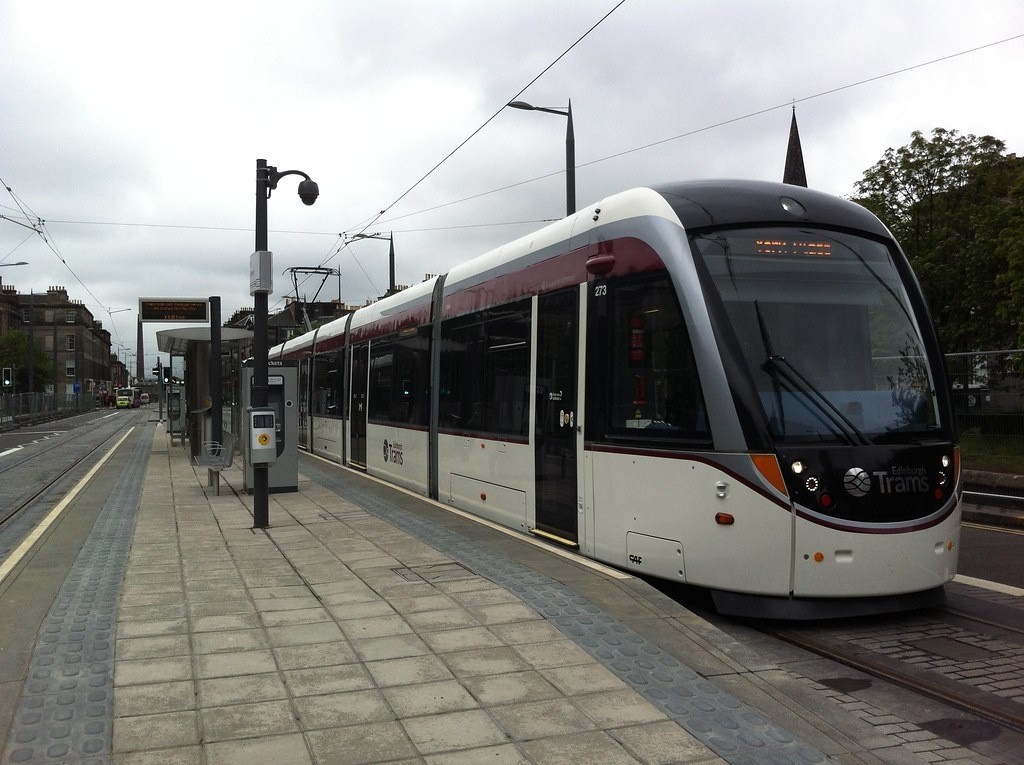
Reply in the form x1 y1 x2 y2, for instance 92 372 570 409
190 406 212 466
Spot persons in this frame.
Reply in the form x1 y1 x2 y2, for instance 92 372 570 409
103 388 116 407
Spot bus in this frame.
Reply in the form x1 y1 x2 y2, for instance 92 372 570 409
244 178 965 623
116 388 141 408
141 394 150 404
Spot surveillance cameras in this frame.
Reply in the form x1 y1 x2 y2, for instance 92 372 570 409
297 181 319 207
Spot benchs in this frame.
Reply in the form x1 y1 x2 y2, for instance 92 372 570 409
194 430 237 496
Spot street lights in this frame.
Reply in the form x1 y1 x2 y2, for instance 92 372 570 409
281 296 307 329
118 347 130 387
352 234 396 295
511 101 577 220
